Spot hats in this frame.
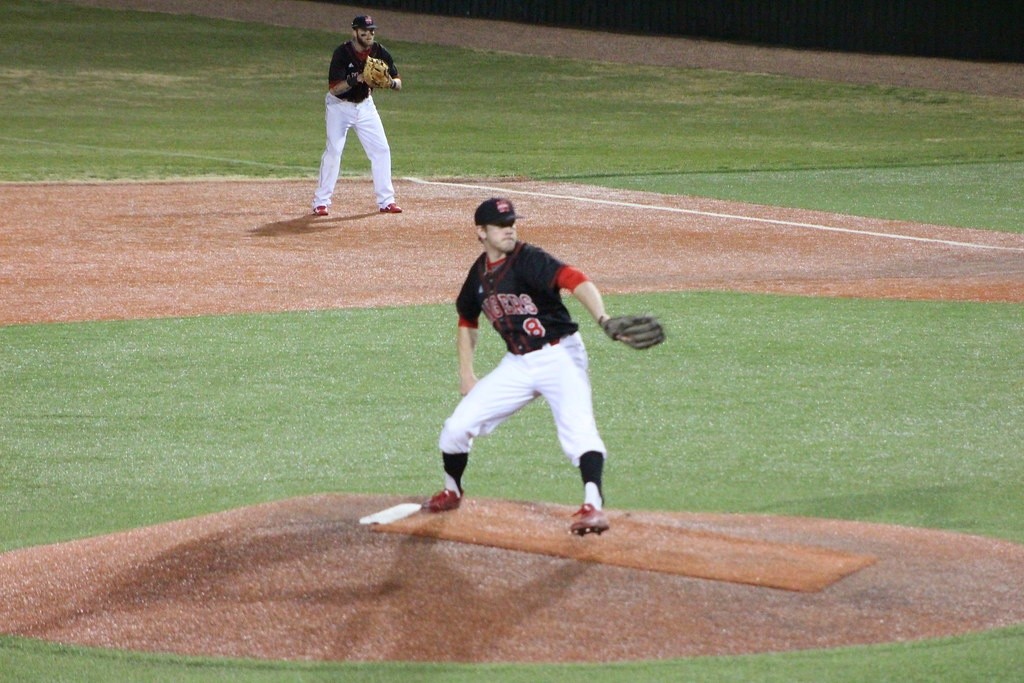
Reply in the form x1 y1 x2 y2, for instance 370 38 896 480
352 15 378 29
474 198 524 225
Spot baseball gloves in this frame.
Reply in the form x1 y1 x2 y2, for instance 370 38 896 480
364 55 390 90
601 316 666 350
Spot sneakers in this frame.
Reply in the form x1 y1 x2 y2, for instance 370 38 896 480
421 487 467 513
571 505 611 536
313 206 328 215
380 204 402 213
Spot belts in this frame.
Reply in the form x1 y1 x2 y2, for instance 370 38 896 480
336 94 371 103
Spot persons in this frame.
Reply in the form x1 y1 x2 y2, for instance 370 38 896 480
312 14 403 213
424 198 666 539
